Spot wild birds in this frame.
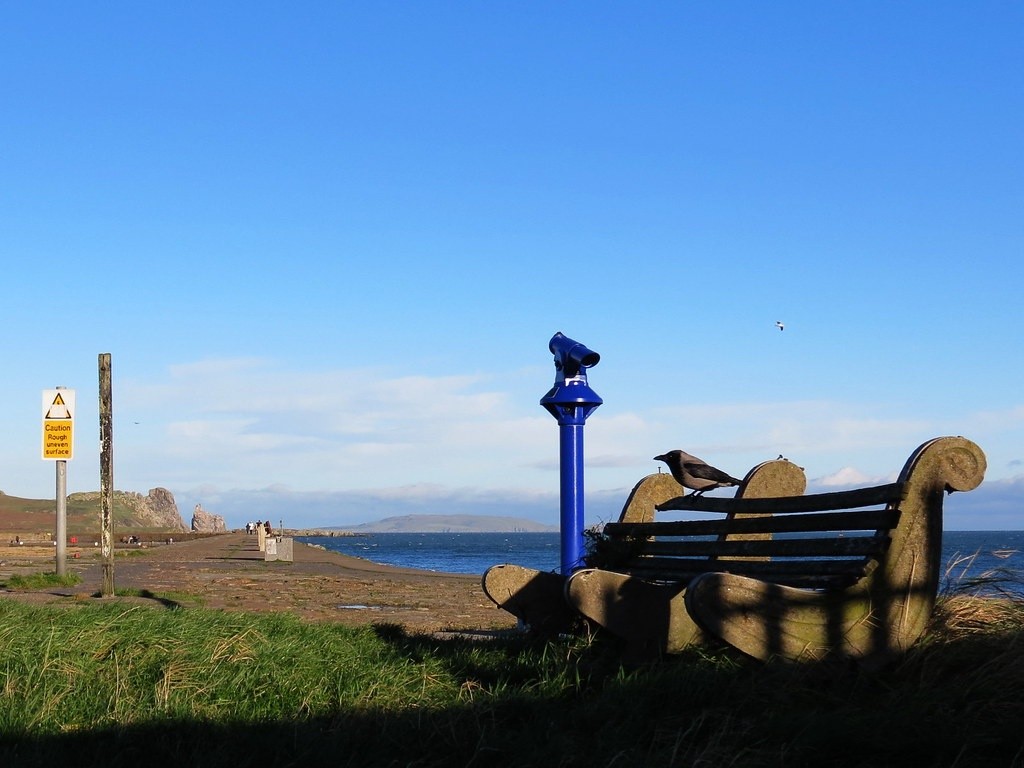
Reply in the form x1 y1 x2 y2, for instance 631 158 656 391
774 321 784 332
653 449 743 497
133 422 139 425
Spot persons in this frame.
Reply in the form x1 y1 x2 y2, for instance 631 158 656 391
165 537 172 545
16 535 20 544
120 535 139 544
245 520 262 535
